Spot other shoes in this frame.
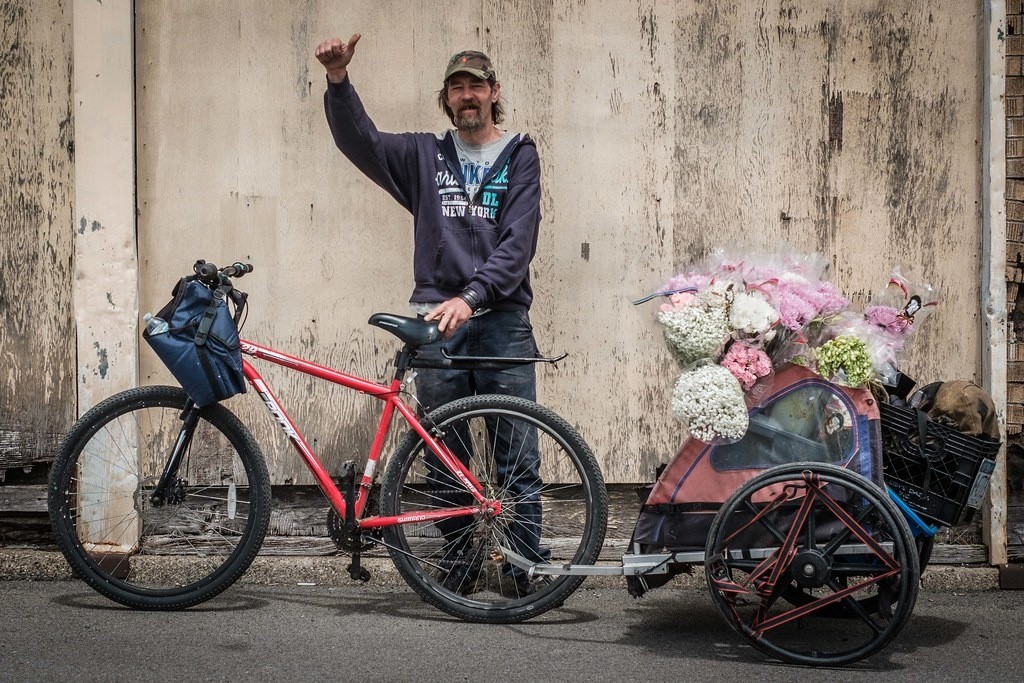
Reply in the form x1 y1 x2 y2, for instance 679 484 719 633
515 576 565 607
432 567 486 598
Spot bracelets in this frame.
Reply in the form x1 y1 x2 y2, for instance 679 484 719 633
460 288 481 310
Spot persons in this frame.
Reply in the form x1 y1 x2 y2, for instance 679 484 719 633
315 32 563 607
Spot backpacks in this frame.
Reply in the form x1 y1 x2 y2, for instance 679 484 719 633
925 380 1000 443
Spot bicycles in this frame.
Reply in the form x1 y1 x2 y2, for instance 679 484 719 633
47 258 612 624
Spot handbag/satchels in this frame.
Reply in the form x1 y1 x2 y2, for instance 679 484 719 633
143 277 246 408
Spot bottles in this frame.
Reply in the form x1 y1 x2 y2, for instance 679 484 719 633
142 313 169 336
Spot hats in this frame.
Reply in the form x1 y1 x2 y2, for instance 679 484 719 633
444 50 495 80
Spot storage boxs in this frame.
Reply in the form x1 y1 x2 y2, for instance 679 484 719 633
879 401 1002 528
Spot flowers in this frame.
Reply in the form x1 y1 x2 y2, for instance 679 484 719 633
633 244 936 446
